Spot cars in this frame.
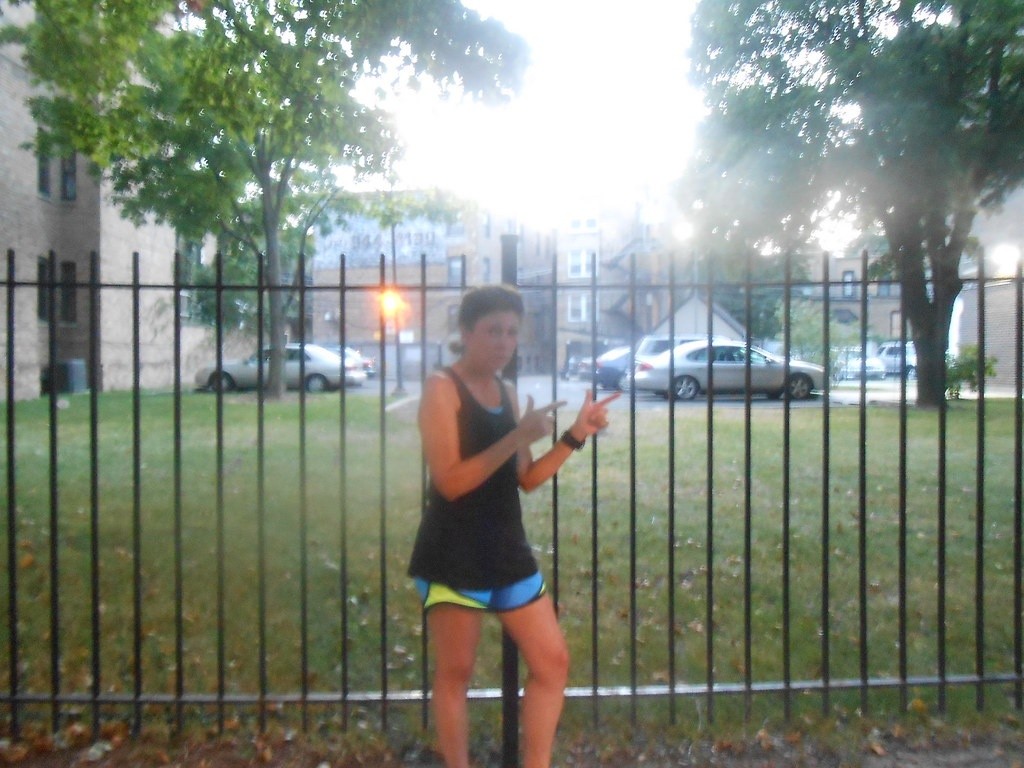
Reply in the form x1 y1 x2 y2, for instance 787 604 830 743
322 344 377 378
829 339 920 383
192 341 365 396
555 332 825 403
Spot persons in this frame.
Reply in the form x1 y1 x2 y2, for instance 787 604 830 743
407 283 620 768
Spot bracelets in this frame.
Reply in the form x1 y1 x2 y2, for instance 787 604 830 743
561 430 585 452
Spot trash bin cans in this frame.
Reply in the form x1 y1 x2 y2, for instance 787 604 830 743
45 359 88 393
